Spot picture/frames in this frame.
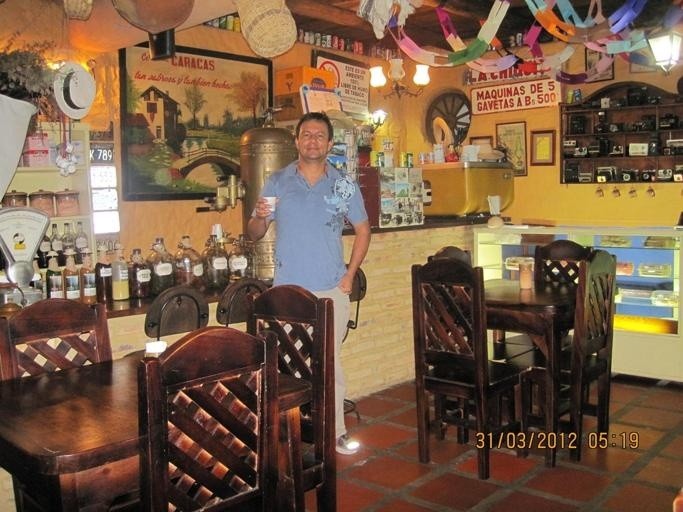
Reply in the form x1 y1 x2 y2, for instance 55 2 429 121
628 41 657 74
118 43 273 202
585 44 615 82
471 136 493 147
530 130 556 166
311 49 371 122
496 121 527 176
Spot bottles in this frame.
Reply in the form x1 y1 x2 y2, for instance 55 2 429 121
519 264 533 290
35 221 253 304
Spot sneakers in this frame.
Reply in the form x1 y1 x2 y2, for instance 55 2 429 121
335 434 360 455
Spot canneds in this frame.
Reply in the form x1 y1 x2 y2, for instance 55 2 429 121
376 152 384 167
398 152 407 167
418 152 425 164
426 152 433 164
407 152 414 167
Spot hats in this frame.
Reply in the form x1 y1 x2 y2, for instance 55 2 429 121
54 62 96 120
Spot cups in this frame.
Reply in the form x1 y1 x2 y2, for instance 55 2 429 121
263 196 276 220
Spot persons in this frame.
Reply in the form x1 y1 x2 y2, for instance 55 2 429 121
247 113 372 456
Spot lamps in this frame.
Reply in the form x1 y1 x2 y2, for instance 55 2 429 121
370 0 431 100
647 22 683 75
369 109 387 134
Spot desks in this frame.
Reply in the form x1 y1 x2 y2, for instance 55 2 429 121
0 350 312 512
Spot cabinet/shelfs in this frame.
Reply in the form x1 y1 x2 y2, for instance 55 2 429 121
559 80 683 183
0 123 96 284
475 225 682 382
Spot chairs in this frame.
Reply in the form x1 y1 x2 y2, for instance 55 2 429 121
0 299 115 381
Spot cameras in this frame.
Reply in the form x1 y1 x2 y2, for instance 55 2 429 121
640 169 656 181
632 114 656 131
659 113 678 129
658 169 672 182
621 168 639 181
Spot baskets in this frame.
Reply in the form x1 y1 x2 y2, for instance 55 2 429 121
235 0 297 58
